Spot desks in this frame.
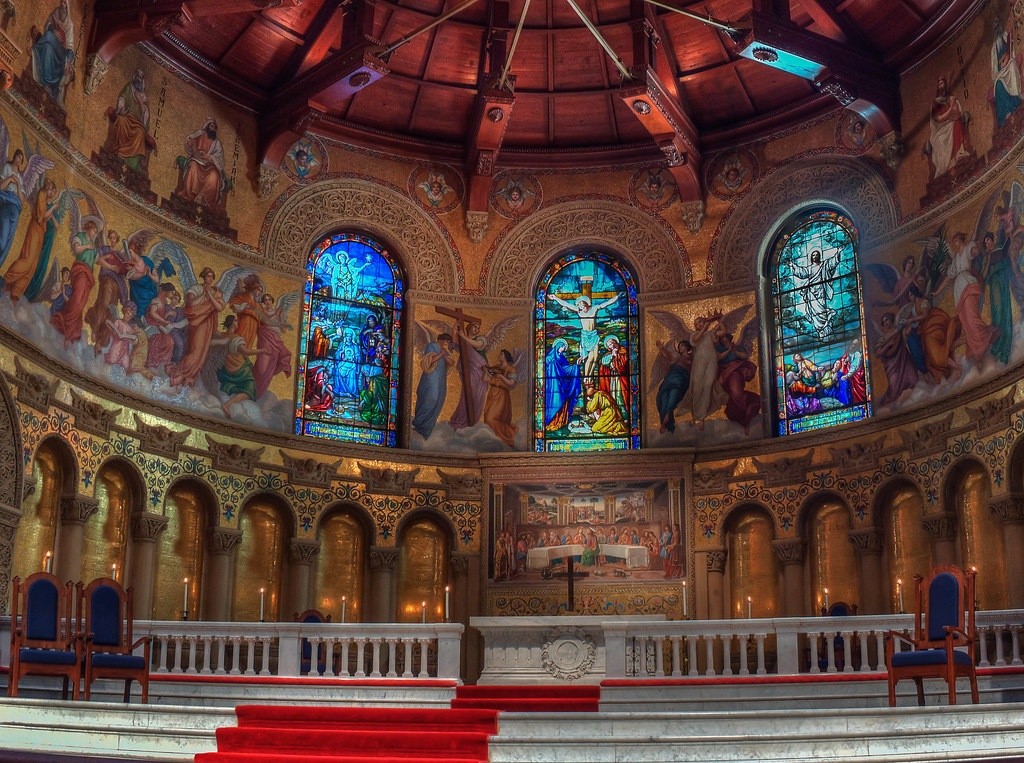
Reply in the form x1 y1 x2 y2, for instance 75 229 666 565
470 614 666 686
527 544 649 571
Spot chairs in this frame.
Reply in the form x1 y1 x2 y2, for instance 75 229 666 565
8 572 83 700
800 601 858 672
882 567 979 708
293 609 341 676
74 577 151 704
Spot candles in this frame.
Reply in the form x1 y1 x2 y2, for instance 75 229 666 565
445 586 449 618
184 578 188 616
898 579 903 610
422 602 426 622
971 566 978 605
46 551 50 573
682 581 687 615
748 596 752 618
825 588 828 611
260 588 264 620
112 564 115 580
342 596 345 622
